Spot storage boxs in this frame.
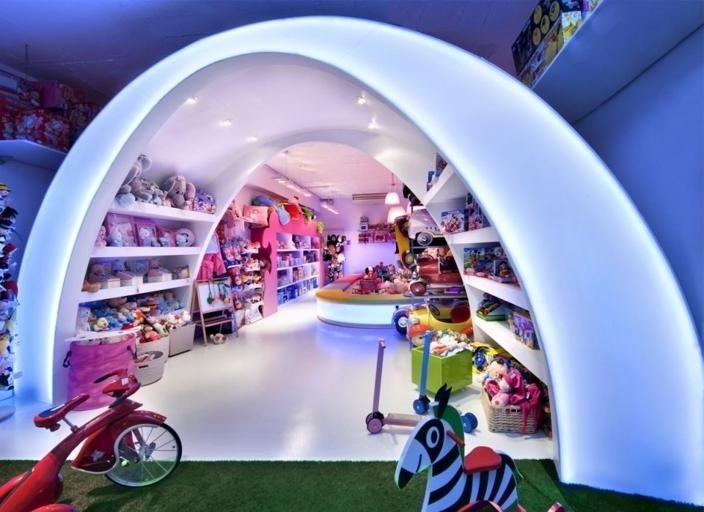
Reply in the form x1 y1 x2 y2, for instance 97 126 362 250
511 0 599 88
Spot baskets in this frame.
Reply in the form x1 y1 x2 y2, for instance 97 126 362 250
480 387 539 434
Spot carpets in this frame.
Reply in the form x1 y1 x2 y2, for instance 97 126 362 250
0 457 702 512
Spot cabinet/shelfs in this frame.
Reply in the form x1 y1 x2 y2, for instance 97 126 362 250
418 161 548 388
73 197 321 357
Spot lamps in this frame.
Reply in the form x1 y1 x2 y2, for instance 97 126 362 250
384 172 406 223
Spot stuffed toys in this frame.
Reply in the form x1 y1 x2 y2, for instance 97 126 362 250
0 182 19 391
422 329 540 411
75 154 217 344
393 153 552 438
